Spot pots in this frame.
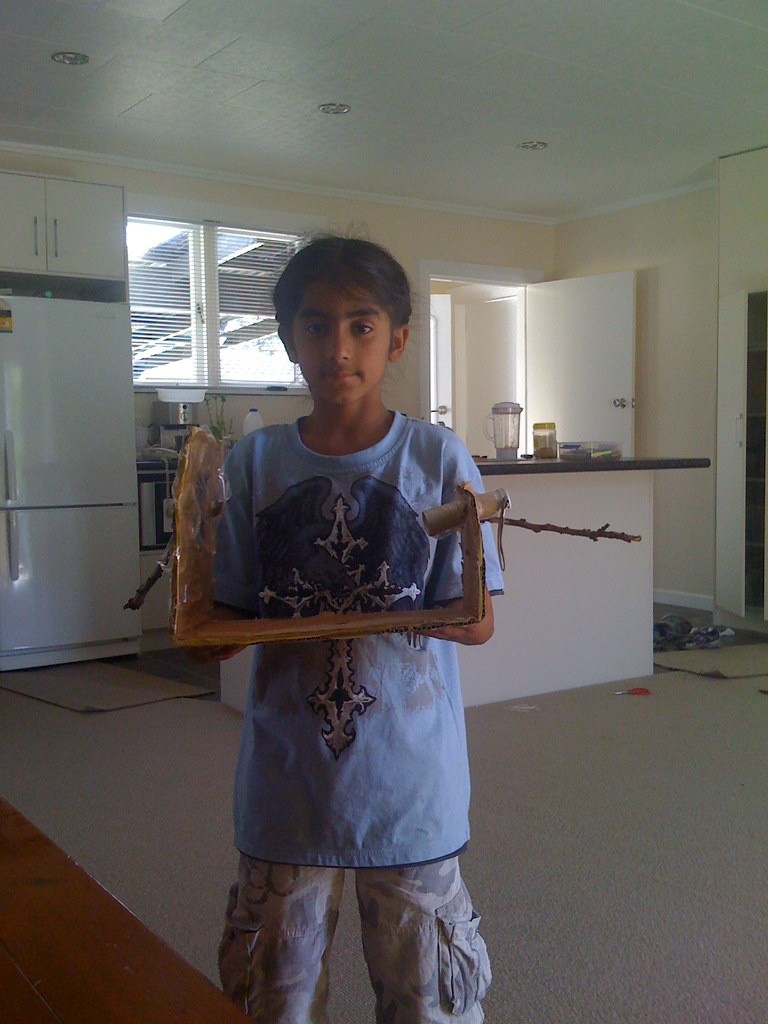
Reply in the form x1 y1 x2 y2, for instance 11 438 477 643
152 401 200 425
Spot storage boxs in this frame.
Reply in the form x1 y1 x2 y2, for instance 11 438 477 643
557 441 622 461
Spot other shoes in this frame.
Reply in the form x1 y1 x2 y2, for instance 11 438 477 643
653 620 674 650
677 626 719 650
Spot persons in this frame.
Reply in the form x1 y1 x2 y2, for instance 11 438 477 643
180 234 505 1024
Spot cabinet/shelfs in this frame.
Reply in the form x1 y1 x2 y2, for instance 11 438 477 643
0 169 130 304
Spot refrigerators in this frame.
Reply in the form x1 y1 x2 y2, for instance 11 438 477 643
0 168 143 671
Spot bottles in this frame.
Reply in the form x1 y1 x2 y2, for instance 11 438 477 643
242 408 264 439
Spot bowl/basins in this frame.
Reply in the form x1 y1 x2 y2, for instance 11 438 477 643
155 388 208 403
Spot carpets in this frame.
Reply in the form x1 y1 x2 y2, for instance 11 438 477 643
654 644 768 679
0 661 217 713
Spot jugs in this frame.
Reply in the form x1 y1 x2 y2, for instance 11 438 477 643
483 402 524 460
